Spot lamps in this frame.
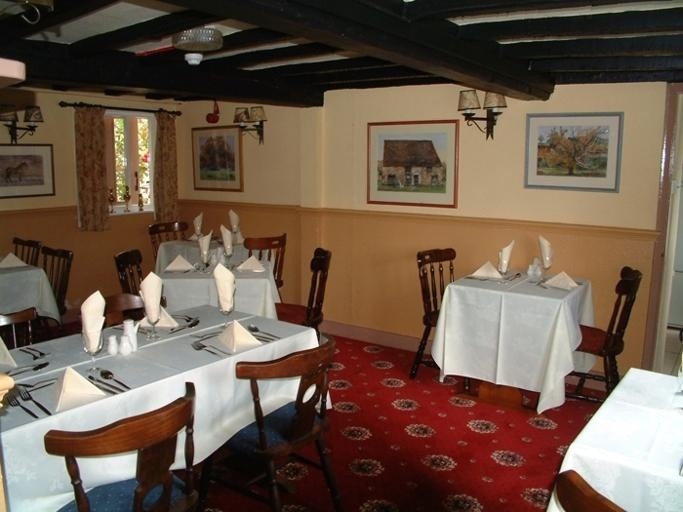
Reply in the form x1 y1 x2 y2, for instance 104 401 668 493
234 106 267 145
0 104 44 144
458 90 507 141
172 25 223 52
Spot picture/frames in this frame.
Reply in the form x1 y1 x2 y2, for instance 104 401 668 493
191 125 244 193
367 120 459 208
524 112 623 194
0 143 55 198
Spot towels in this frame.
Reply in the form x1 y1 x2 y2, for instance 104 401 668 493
544 272 577 291
539 234 551 265
194 211 204 233
467 260 502 279
501 239 514 272
228 208 239 230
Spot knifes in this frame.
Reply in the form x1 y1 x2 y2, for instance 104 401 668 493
88 375 125 393
193 335 220 343
26 382 55 393
112 325 156 335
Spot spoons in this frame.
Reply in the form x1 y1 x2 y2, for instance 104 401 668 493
8 362 48 376
168 322 197 334
509 273 520 282
247 323 282 340
463 276 487 282
99 369 131 390
15 377 57 387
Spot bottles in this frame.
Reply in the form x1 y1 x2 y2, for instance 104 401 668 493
108 334 118 355
119 336 131 355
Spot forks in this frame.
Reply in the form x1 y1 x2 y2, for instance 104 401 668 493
18 388 52 415
169 314 198 323
5 393 38 419
191 341 231 358
19 346 50 360
195 331 222 338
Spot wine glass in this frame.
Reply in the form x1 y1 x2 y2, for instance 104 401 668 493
144 308 160 340
80 333 103 373
498 255 509 284
217 301 233 328
200 251 210 273
231 225 239 245
223 247 233 269
541 255 552 279
192 224 201 244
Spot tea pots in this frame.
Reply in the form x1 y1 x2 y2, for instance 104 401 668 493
123 319 140 352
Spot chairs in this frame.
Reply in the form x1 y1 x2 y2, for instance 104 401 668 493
409 248 471 393
565 266 642 404
556 470 626 512
